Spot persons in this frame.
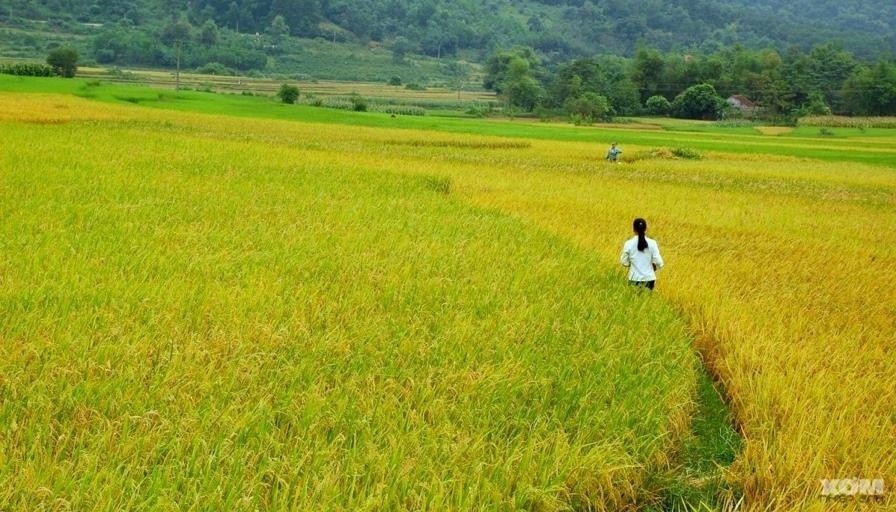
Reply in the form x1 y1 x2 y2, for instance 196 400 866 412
606 141 622 162
620 217 664 289
